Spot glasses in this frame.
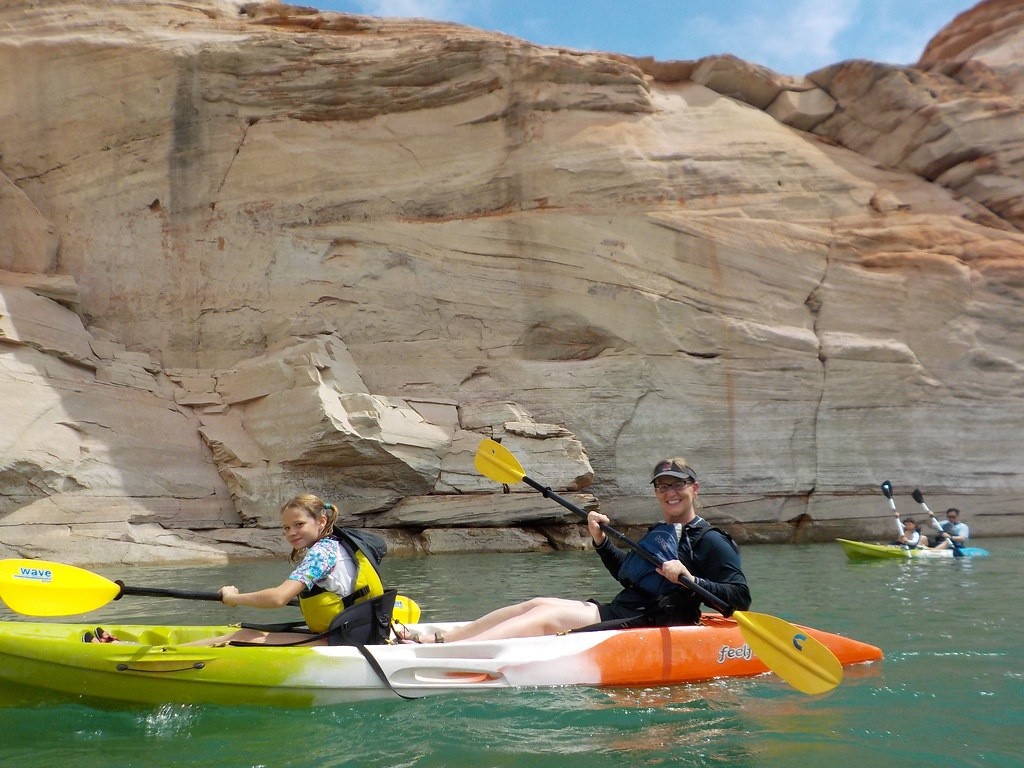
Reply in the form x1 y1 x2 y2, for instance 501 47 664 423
946 515 957 519
655 480 691 493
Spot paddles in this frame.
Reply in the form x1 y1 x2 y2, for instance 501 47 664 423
475 438 843 694
881 480 912 558
0 558 425 626
911 488 965 557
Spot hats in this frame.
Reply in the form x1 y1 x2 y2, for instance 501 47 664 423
649 463 696 484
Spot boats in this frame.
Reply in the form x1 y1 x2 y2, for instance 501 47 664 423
836 538 990 559
0 613 886 706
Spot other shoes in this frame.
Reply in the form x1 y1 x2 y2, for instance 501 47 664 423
82 627 118 645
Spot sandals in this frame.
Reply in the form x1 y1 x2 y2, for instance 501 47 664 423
393 627 445 645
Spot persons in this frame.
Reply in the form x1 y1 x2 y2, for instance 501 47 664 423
917 508 969 550
80 493 384 646
390 457 754 644
875 511 919 548
